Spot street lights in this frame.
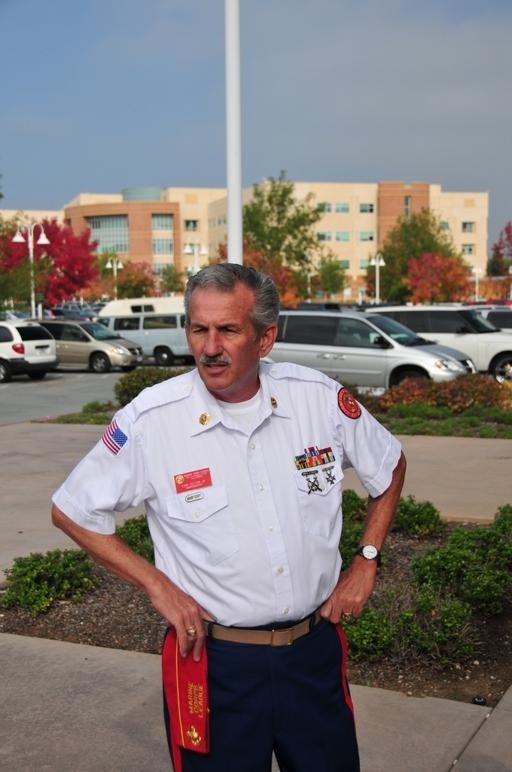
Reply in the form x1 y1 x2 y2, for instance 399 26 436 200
184 235 209 276
470 265 484 302
105 254 125 298
12 218 50 319
370 252 387 306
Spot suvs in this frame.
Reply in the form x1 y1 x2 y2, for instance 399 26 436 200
0 320 61 384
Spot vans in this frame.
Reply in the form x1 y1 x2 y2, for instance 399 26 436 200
39 320 144 373
95 312 199 367
368 305 512 388
96 296 186 314
262 310 477 395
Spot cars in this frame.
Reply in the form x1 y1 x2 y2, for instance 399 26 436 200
477 307 512 332
0 299 107 323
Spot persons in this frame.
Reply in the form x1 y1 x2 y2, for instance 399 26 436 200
50 263 406 772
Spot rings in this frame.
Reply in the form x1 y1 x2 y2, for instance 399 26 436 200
343 613 354 624
185 626 197 638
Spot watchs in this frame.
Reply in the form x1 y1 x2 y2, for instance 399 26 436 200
354 545 383 566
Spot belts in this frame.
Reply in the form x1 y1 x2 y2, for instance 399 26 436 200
182 609 334 649
